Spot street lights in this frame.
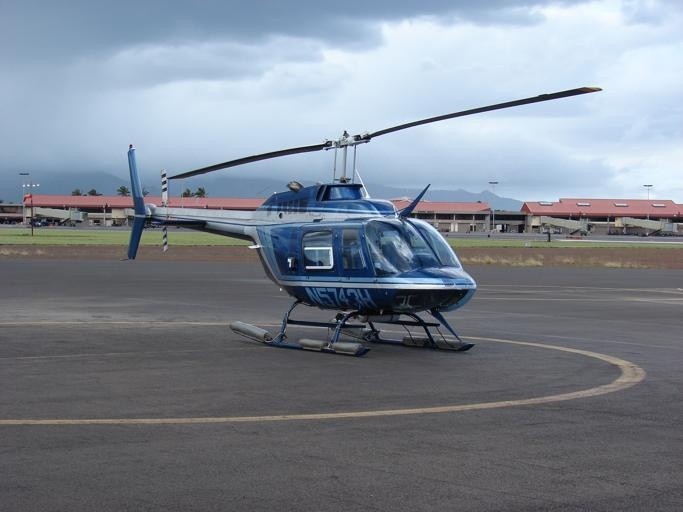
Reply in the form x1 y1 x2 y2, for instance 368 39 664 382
18 172 30 226
642 184 653 236
21 182 40 235
487 180 498 233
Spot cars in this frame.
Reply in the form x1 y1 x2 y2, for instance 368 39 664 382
143 224 155 229
33 220 42 227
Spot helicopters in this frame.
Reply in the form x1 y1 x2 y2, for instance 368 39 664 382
124 86 604 356
